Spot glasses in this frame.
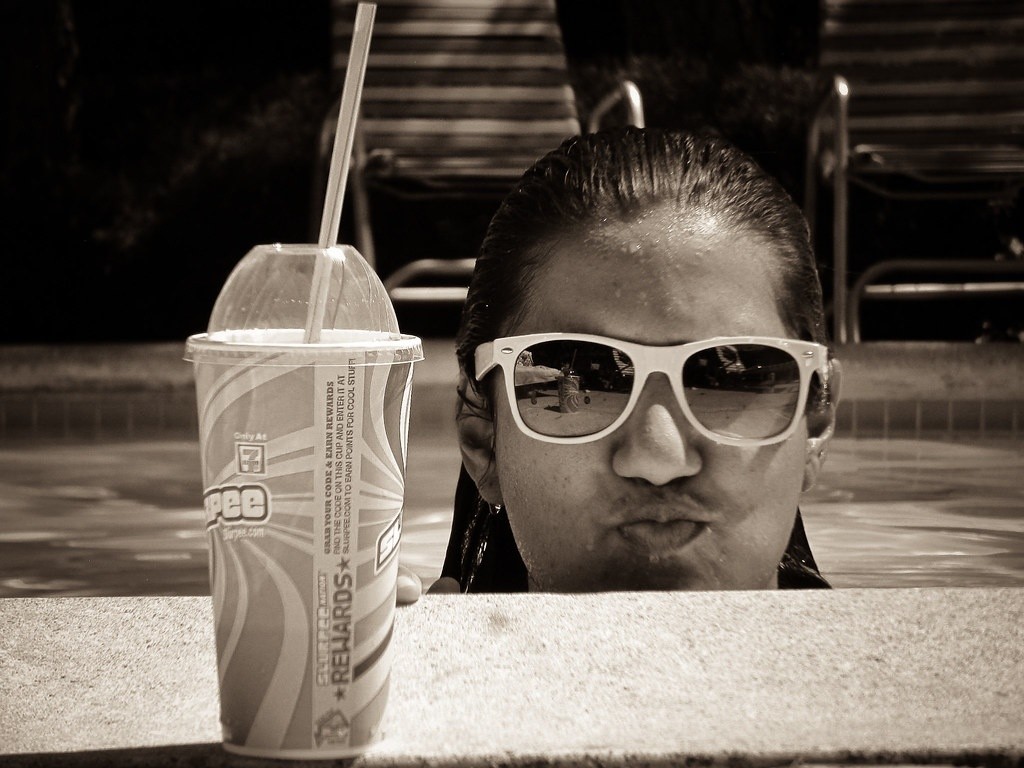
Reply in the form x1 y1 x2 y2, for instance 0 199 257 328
475 334 827 445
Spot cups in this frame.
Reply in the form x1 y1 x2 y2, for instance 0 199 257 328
556 367 580 414
188 244 422 756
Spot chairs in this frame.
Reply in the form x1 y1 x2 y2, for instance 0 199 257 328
322 0 648 302
514 352 563 404
807 0 1024 343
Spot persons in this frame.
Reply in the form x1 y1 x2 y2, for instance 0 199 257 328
396 125 843 603
